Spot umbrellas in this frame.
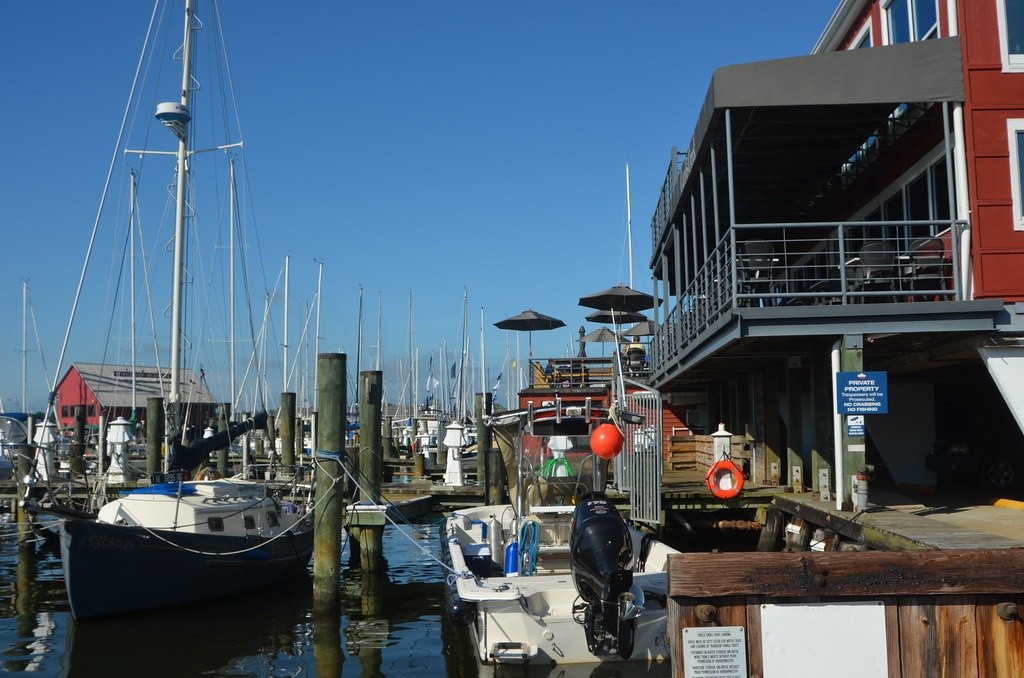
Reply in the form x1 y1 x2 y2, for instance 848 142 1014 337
577 326 586 386
492 308 568 358
575 327 631 382
578 281 663 342
623 320 662 367
584 310 648 332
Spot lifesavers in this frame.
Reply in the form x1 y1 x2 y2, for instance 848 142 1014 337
412 440 417 451
705 460 745 500
196 466 209 480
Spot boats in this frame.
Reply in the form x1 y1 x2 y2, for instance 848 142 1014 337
447 488 683 678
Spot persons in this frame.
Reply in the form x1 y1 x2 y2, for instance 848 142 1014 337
544 359 558 387
626 336 644 352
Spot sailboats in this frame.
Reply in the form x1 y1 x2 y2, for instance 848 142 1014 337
1 0 528 629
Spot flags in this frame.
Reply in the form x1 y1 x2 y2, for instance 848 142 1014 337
427 357 439 391
451 362 455 378
513 362 515 369
492 373 502 402
